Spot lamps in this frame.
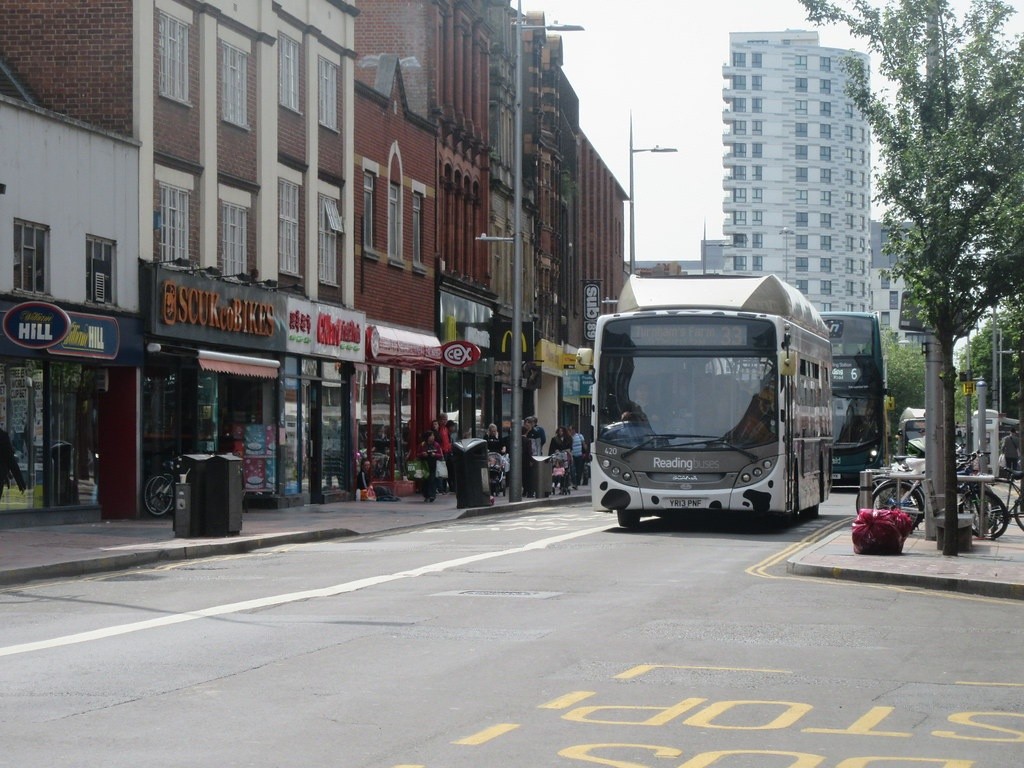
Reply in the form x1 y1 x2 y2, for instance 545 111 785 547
147 257 304 293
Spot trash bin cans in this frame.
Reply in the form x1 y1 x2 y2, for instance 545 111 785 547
531 455 553 499
49 440 79 505
453 439 491 509
173 453 242 537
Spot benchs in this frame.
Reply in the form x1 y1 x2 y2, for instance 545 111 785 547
922 478 974 552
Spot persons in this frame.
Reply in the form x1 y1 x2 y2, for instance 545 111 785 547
1001 428 1019 470
548 426 573 456
0 429 27 502
568 425 590 490
621 384 658 420
357 458 370 490
416 413 458 505
522 416 546 498
482 423 506 455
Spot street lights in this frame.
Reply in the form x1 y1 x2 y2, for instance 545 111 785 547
474 0 587 503
779 226 796 284
629 107 679 277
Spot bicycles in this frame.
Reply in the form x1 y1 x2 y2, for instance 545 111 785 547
142 455 190 518
856 429 1024 541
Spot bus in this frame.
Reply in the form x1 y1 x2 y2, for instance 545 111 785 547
574 274 834 535
894 406 926 464
816 310 896 492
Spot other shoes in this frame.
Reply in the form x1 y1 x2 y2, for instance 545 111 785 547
424 497 431 503
572 484 578 490
523 489 527 496
527 491 534 497
430 496 435 502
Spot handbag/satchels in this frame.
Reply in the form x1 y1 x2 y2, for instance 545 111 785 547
435 460 448 480
406 457 430 482
999 454 1006 467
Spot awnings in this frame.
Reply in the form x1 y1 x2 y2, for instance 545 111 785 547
158 343 280 379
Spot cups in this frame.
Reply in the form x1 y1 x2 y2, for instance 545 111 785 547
180 474 186 483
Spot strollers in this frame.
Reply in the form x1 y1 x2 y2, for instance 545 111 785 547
487 450 510 497
550 450 573 495
